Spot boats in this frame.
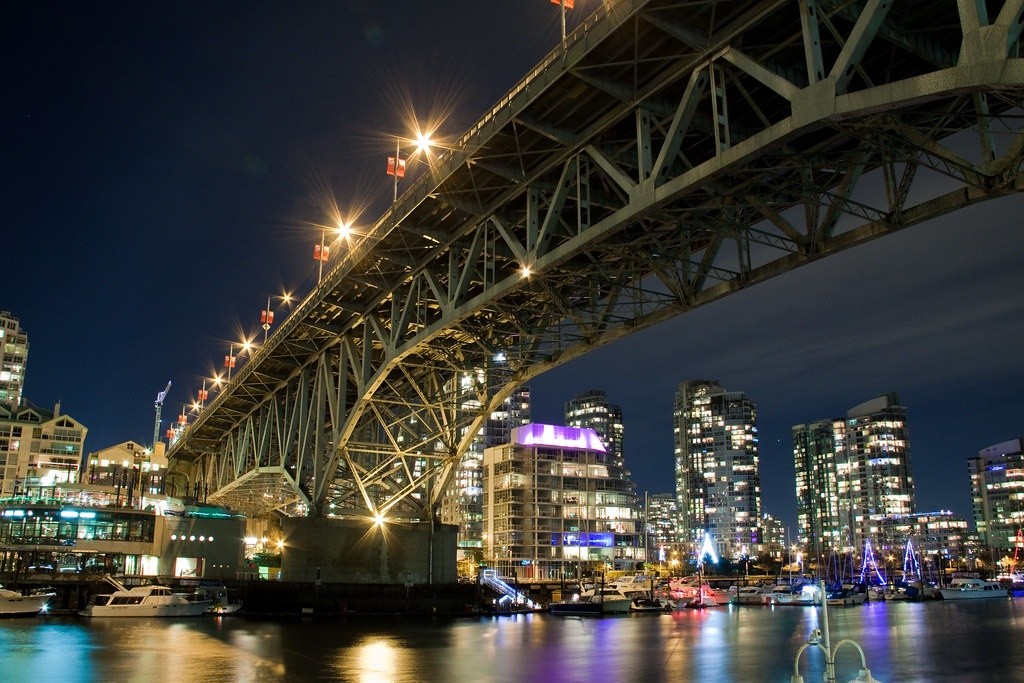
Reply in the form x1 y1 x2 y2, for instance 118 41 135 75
0 584 57 618
76 573 217 618
173 586 245 615
932 578 1009 599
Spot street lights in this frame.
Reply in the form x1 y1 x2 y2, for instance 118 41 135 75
393 135 431 200
318 228 349 284
264 296 289 342
182 405 199 435
169 422 185 449
228 343 251 381
201 378 221 411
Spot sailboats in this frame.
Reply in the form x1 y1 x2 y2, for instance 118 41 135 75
471 490 939 617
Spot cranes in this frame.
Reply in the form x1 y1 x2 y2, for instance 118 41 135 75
153 380 173 443
